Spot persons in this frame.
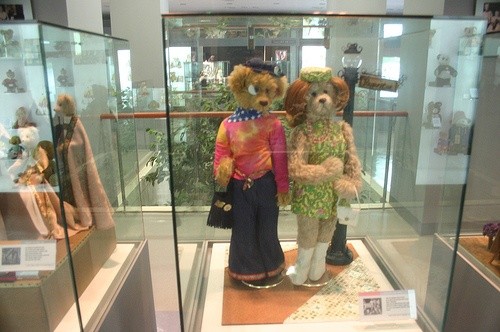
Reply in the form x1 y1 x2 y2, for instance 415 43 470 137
448 111 472 146
482 4 500 32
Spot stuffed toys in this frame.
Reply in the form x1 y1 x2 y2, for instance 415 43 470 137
8 136 26 159
213 58 289 280
2 69 18 92
14 94 115 230
425 101 443 130
435 53 457 87
284 66 363 286
12 107 37 130
463 26 481 54
0 28 21 57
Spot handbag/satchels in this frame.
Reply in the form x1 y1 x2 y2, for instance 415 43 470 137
206 179 233 230
336 184 361 225
